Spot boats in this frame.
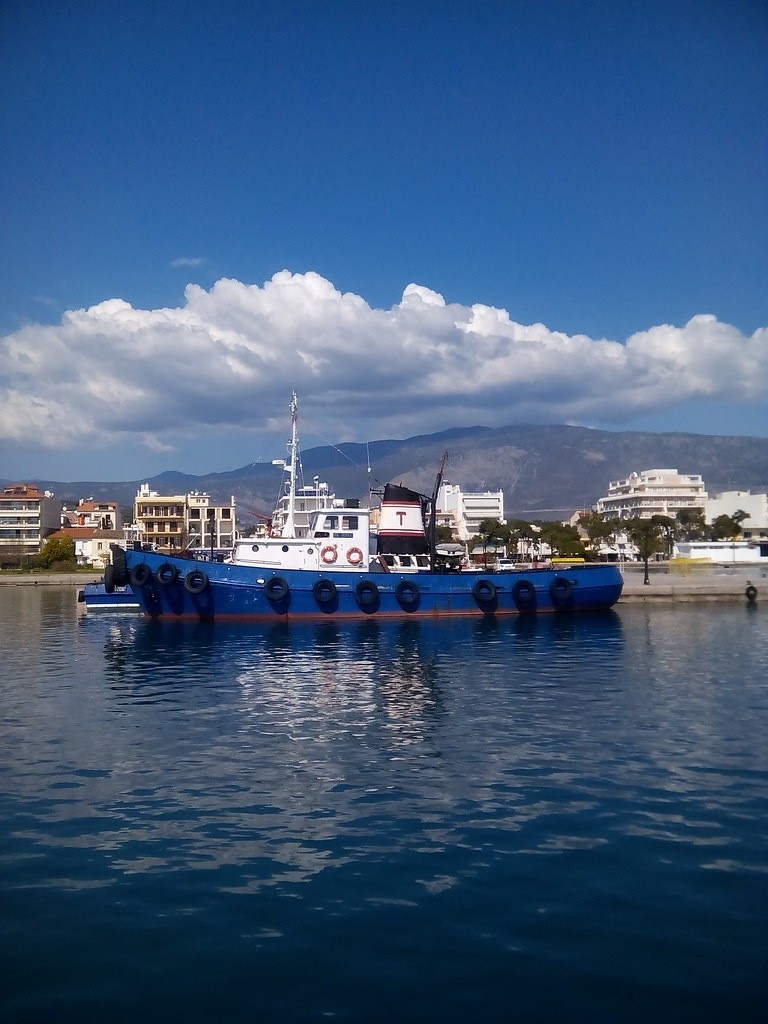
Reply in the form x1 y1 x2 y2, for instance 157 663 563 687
102 389 624 622
78 577 139 613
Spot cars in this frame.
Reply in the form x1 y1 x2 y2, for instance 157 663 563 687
493 558 515 571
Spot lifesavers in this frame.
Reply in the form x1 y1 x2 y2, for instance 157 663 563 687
154 564 180 585
353 581 379 606
113 547 127 586
347 547 363 564
184 570 208 594
264 577 289 601
105 564 115 594
130 563 151 586
549 577 574 601
313 579 338 603
745 586 757 600
474 580 496 604
395 580 421 605
320 546 337 563
512 579 537 604
